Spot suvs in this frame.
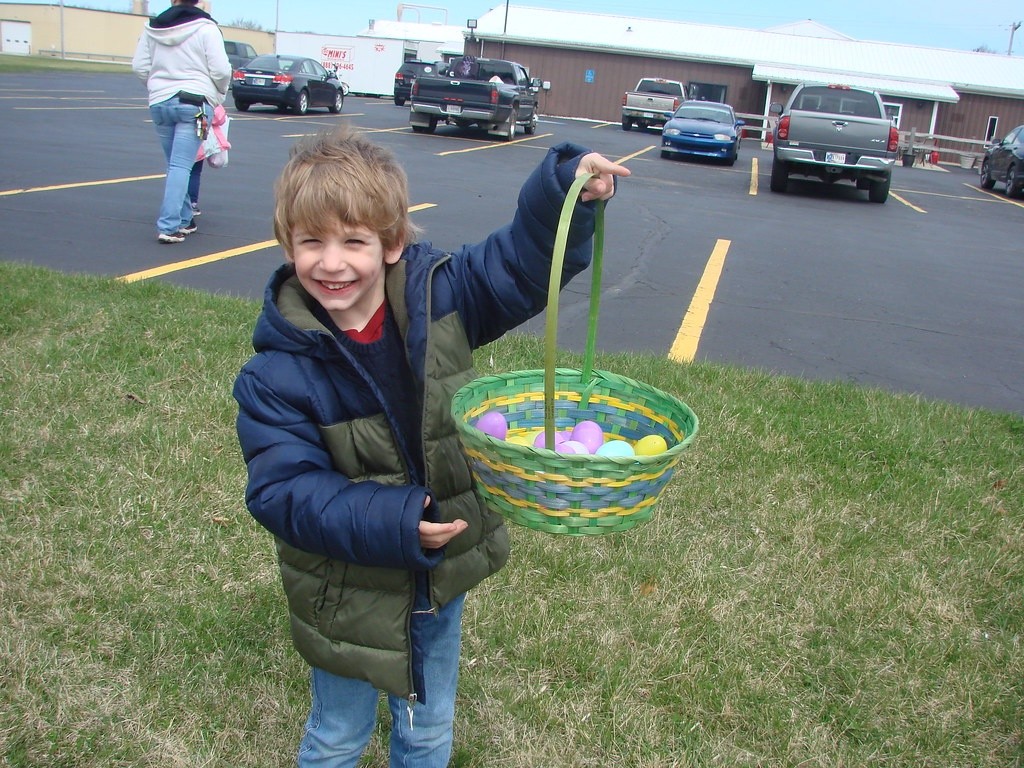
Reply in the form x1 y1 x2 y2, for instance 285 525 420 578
394 60 450 106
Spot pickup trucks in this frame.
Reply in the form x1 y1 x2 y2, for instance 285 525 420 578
768 81 900 203
622 78 689 131
409 56 542 141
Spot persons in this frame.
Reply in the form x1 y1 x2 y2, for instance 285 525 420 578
187 0 212 217
131 0 233 243
231 122 633 767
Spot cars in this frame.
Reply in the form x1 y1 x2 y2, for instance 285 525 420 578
981 124 1024 197
661 100 746 167
232 55 344 115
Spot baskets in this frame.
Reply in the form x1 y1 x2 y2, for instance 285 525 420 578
450 169 702 537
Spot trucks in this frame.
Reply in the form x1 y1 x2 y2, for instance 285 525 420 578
223 30 405 98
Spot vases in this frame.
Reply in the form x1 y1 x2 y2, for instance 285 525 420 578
977 158 984 174
960 155 976 169
902 154 916 168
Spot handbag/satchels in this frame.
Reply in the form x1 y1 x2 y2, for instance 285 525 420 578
207 117 230 169
212 105 226 126
193 120 231 163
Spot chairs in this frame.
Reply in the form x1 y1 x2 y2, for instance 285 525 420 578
801 98 820 110
282 66 291 71
853 103 873 115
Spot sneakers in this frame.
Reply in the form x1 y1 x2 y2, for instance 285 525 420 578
179 220 197 235
158 233 185 243
188 204 201 216
